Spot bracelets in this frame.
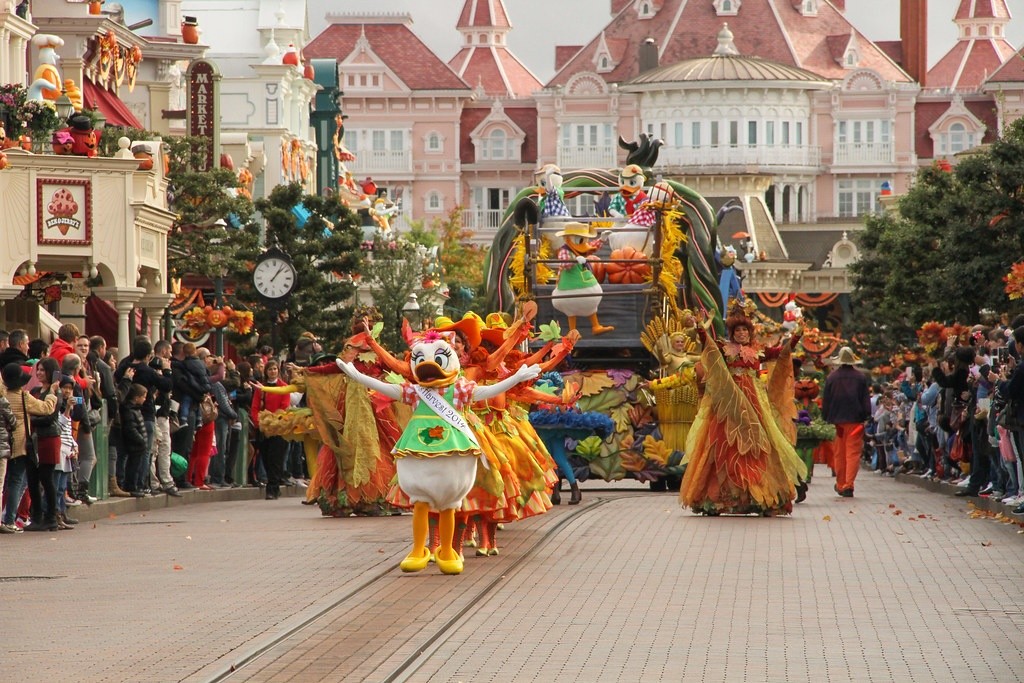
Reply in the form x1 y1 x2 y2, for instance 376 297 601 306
995 376 999 383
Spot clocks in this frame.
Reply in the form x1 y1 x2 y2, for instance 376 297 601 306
254 258 293 299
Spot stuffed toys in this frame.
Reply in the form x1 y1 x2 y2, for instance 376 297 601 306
349 164 673 572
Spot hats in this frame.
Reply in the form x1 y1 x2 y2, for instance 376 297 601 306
2 363 32 390
725 303 755 347
830 346 863 364
427 311 513 355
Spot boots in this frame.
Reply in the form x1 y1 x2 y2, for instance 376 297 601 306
794 473 808 503
551 481 582 504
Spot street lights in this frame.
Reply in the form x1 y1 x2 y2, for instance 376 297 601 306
187 218 251 357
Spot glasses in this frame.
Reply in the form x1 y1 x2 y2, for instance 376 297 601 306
207 354 212 358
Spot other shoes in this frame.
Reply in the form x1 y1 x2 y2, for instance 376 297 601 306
429 553 465 563
0 507 78 533
65 482 97 506
834 483 854 497
462 531 479 547
476 547 499 557
109 474 243 496
250 477 311 499
954 482 1024 515
874 464 970 487
496 522 505 530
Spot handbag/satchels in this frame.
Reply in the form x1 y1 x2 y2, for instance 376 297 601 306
916 417 930 435
200 394 218 420
995 399 1017 431
67 458 78 498
937 397 968 434
88 386 102 425
27 433 39 469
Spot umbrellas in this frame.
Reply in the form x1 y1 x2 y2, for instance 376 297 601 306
732 232 749 238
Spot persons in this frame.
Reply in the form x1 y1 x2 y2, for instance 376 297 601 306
16 0 29 19
636 309 1024 516
0 324 407 534
740 237 756 255
